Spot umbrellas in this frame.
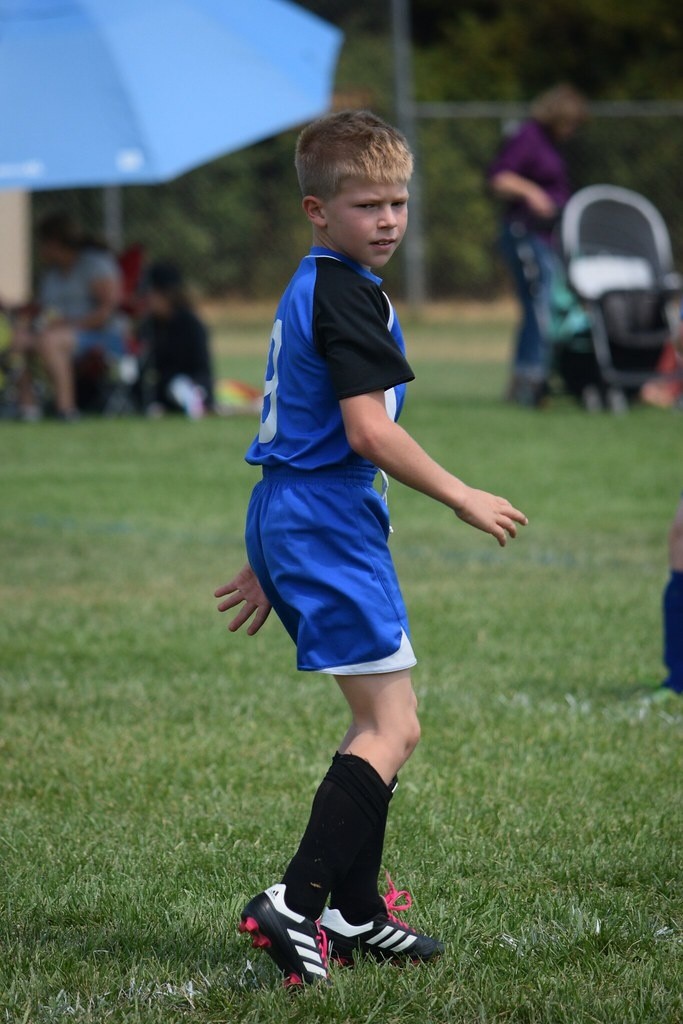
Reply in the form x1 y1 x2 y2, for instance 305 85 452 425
1 1 344 188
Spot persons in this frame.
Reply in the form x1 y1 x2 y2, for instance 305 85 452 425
482 75 582 404
211 111 530 987
35 208 213 417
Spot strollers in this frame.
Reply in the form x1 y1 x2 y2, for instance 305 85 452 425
538 170 683 405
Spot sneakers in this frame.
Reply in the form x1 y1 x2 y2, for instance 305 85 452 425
320 870 445 970
238 883 331 999
